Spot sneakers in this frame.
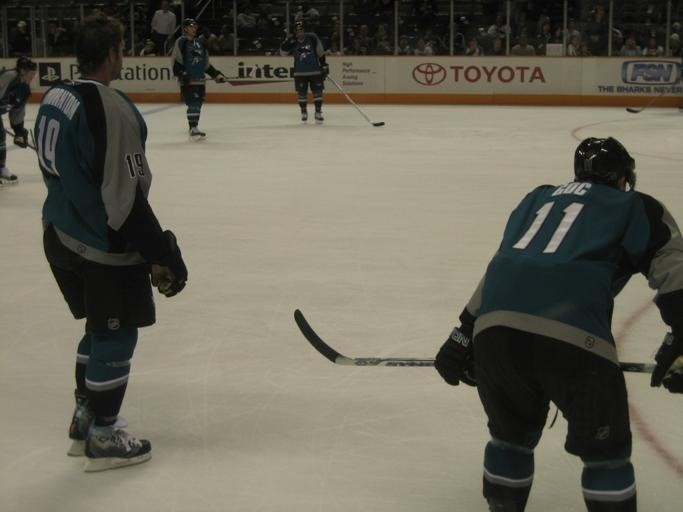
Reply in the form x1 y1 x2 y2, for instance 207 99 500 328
0 168 17 181
187 128 205 137
314 110 323 120
67 391 97 439
301 112 308 121
84 425 150 458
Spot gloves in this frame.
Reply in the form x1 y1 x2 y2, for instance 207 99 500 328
650 331 683 394
12 129 29 149
213 72 226 84
148 229 189 295
433 326 478 387
179 73 190 84
282 35 297 51
321 63 329 78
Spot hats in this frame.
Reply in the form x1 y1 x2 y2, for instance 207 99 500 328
16 20 27 28
672 23 680 28
670 34 680 41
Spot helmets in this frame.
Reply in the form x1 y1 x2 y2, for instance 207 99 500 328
17 56 36 72
572 137 636 192
181 18 196 30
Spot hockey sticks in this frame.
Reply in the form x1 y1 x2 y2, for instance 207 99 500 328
188 66 258 82
327 75 384 126
627 72 683 113
293 309 657 373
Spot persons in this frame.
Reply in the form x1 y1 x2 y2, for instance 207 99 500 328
435 137 683 512
8 1 683 58
34 10 187 460
279 22 329 122
171 18 228 136
1 56 38 181
